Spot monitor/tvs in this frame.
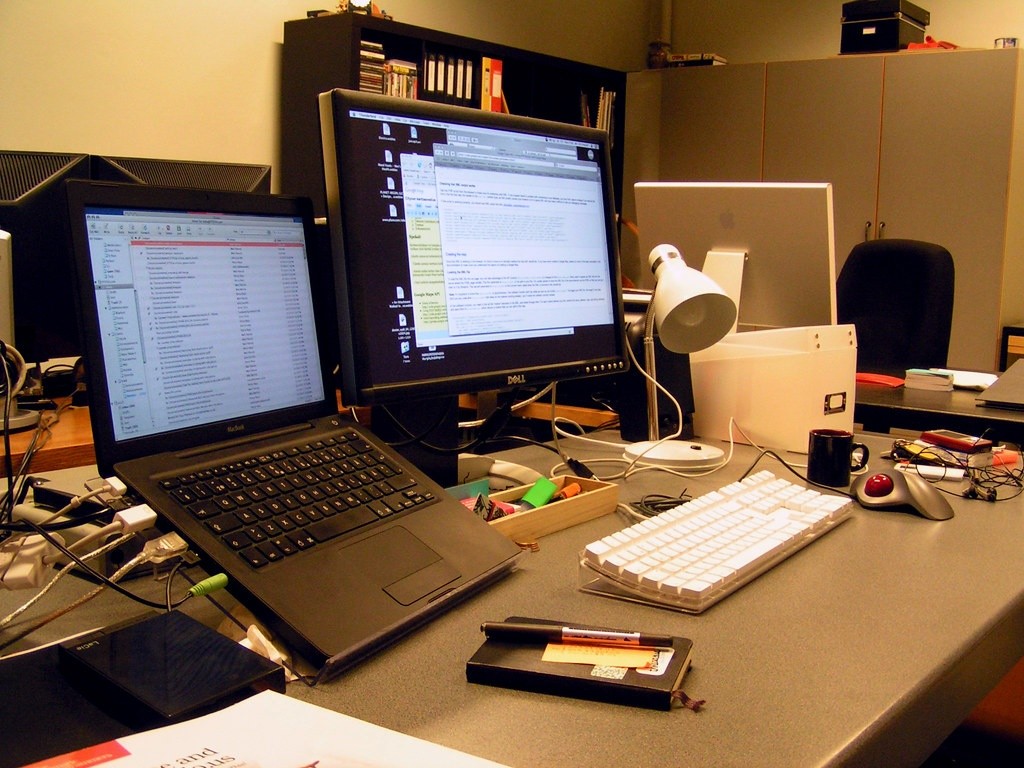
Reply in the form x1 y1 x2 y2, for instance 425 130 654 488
0 149 272 400
319 88 631 493
632 182 837 329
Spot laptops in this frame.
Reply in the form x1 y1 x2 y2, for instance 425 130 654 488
67 176 523 671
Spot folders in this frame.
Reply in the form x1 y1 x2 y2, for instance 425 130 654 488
427 53 502 112
974 358 1024 411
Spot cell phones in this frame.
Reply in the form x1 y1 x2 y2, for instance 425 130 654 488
920 429 992 452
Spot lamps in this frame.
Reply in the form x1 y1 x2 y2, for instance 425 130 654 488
623 245 737 467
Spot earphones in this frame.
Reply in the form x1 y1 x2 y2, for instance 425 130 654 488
977 487 997 501
962 484 978 499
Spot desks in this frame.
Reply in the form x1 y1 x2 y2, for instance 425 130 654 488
0 380 1022 768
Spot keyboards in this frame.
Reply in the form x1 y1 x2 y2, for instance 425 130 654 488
577 470 853 615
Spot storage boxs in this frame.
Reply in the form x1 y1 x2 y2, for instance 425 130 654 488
839 0 930 53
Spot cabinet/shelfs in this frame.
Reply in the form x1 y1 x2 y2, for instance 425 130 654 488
280 12 1024 415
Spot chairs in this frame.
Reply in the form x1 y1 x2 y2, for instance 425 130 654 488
834 238 955 379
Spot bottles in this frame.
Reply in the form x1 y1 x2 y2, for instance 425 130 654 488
647 41 670 69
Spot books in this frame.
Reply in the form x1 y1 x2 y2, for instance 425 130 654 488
360 41 417 99
666 54 727 67
905 369 954 391
467 616 692 711
582 87 618 146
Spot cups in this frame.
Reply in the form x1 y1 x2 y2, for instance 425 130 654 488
807 429 869 487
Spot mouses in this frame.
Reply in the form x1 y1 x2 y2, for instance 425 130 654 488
849 469 955 521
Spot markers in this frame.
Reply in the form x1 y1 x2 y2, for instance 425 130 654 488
479 620 674 647
